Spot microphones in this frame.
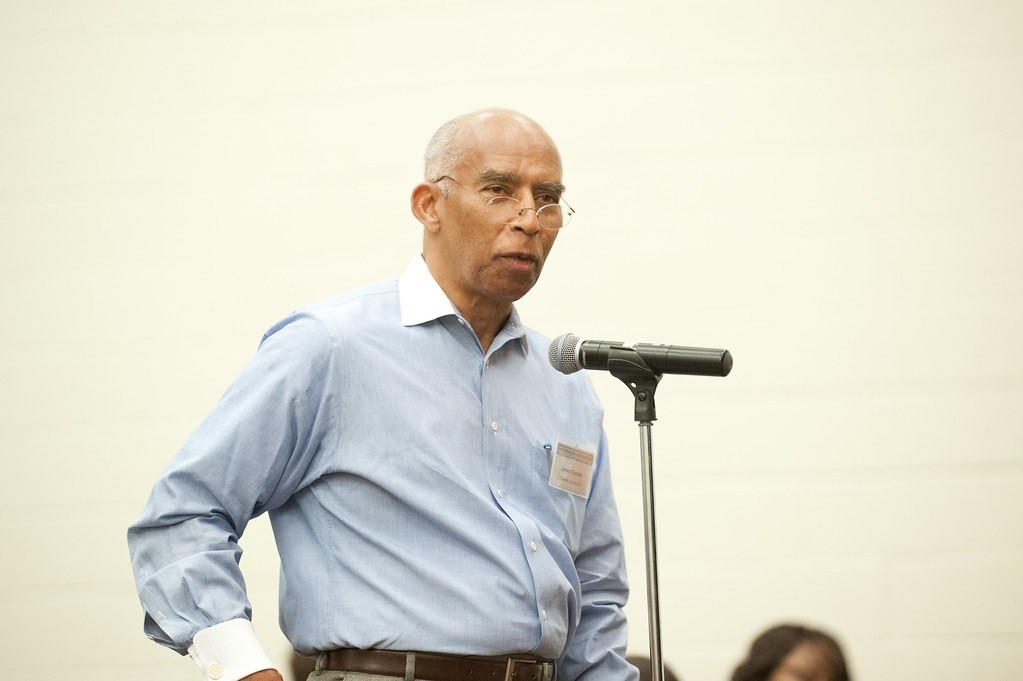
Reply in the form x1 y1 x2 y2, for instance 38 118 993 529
549 332 735 378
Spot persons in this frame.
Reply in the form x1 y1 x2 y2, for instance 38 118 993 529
729 623 850 680
123 108 643 681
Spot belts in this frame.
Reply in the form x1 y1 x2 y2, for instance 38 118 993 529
288 651 566 681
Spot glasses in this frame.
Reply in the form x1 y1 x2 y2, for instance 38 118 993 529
431 175 577 231
